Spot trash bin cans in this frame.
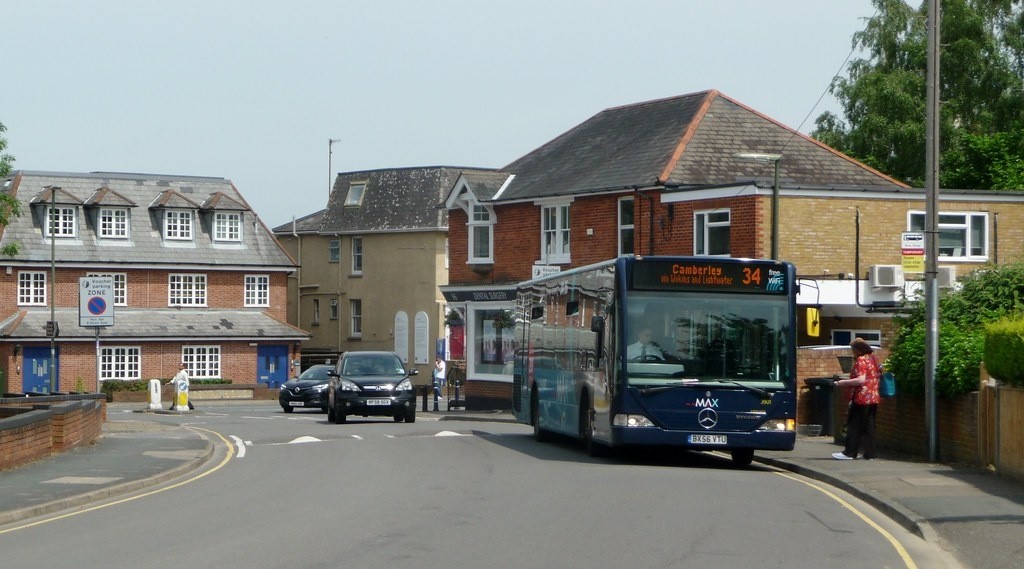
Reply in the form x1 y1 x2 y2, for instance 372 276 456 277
804 377 836 435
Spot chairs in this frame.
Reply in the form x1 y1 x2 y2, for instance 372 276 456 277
704 339 737 378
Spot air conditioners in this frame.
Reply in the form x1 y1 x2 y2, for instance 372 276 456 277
869 264 905 288
938 266 956 288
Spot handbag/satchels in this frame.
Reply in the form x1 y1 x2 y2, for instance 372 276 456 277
872 354 895 397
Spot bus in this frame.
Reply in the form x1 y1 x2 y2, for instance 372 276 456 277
512 254 823 467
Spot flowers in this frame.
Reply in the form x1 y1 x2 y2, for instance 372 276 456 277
442 308 464 336
492 307 514 328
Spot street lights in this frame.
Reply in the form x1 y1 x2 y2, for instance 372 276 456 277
49 186 63 395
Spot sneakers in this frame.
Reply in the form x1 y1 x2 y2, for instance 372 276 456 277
831 452 854 459
855 453 874 461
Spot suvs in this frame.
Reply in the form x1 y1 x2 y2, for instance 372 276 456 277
326 350 420 424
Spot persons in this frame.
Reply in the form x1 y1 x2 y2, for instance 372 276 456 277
434 356 446 400
619 326 665 361
165 363 194 412
831 338 880 461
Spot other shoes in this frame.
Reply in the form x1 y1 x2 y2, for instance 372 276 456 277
168 408 173 410
190 408 194 409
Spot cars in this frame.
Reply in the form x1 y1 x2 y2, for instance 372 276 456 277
279 364 337 413
